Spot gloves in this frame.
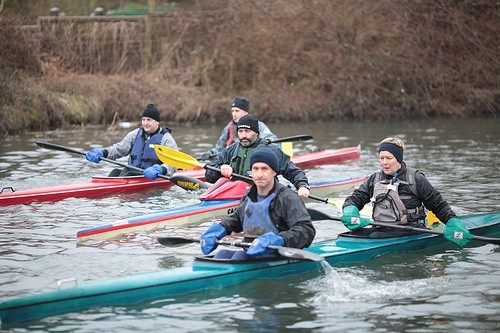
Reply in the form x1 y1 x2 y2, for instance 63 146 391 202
341 205 370 231
200 222 227 255
86 148 104 163
443 218 472 248
143 163 162 180
246 231 285 256
202 151 212 161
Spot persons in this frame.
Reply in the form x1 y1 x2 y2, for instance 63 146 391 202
340 138 473 248
86 104 178 179
201 98 282 161
205 114 311 198
200 148 316 260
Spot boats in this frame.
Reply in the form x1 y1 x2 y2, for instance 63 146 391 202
0 139 363 207
0 209 500 333
75 174 370 244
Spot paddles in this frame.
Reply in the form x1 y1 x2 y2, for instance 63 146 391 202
305 208 500 245
35 140 210 191
197 134 316 161
156 236 324 263
154 144 373 217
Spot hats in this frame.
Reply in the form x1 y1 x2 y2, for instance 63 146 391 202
236 114 260 135
230 97 250 112
142 104 160 122
250 147 279 172
378 137 404 164
